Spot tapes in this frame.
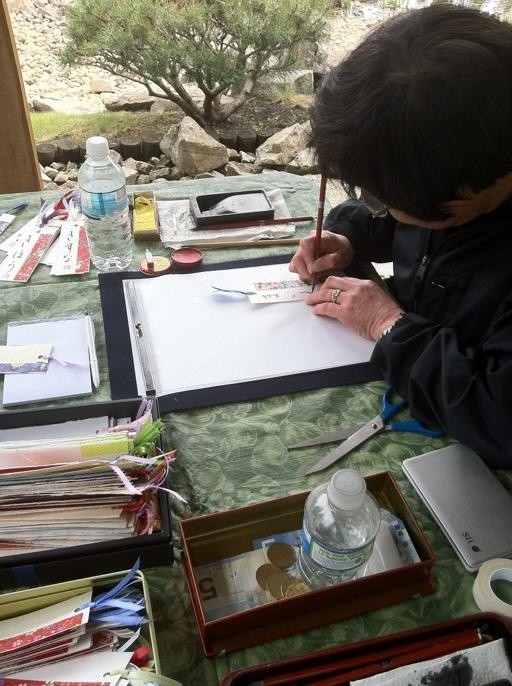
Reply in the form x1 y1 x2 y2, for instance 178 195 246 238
473 558 512 620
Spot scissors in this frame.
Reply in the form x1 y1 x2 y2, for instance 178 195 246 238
286 387 446 476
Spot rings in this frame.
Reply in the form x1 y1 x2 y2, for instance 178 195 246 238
331 288 341 303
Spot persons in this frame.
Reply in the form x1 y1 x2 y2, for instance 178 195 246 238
285 2 511 475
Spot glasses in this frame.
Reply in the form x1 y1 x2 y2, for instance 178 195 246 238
340 177 390 221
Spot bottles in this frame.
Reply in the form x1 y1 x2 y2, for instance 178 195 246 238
79 136 132 271
298 469 383 592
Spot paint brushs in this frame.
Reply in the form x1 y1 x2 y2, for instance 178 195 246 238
189 216 313 231
310 159 328 294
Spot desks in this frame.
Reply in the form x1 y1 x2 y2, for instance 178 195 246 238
0 175 512 686
